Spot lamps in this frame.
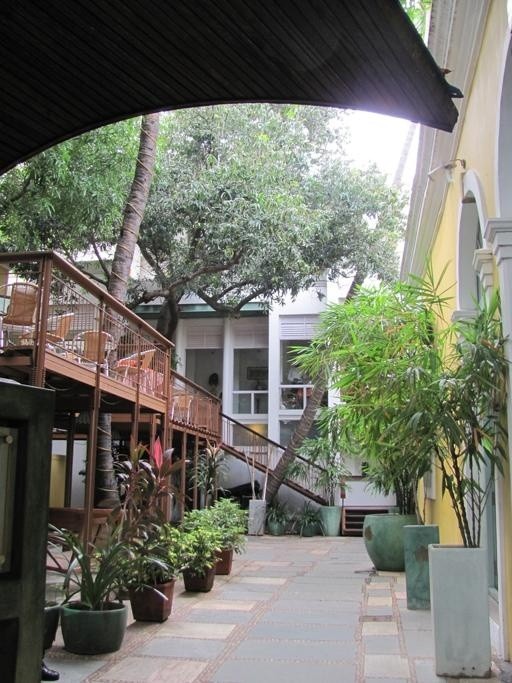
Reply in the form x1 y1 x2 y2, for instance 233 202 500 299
428 156 466 181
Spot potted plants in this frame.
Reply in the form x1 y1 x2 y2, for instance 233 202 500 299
45 440 287 655
287 251 511 682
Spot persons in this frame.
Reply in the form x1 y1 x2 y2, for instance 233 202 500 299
207 373 222 399
288 363 303 410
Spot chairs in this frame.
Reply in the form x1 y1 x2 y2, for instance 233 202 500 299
0 281 195 424
